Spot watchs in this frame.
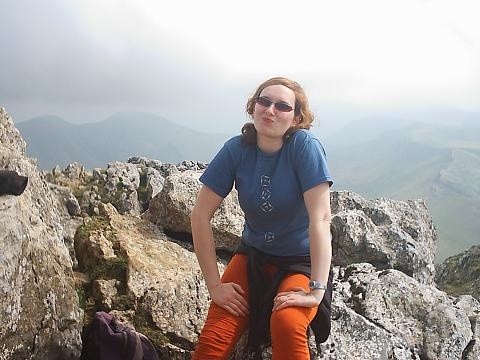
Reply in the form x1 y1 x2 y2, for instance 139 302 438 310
309 280 327 290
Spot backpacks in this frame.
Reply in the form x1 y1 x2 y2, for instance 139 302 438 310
81 312 161 360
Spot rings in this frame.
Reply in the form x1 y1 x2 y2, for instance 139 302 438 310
287 295 291 298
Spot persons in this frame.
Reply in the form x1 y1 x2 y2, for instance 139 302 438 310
190 77 334 359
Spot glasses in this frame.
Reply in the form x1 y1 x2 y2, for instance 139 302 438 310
255 97 295 112
